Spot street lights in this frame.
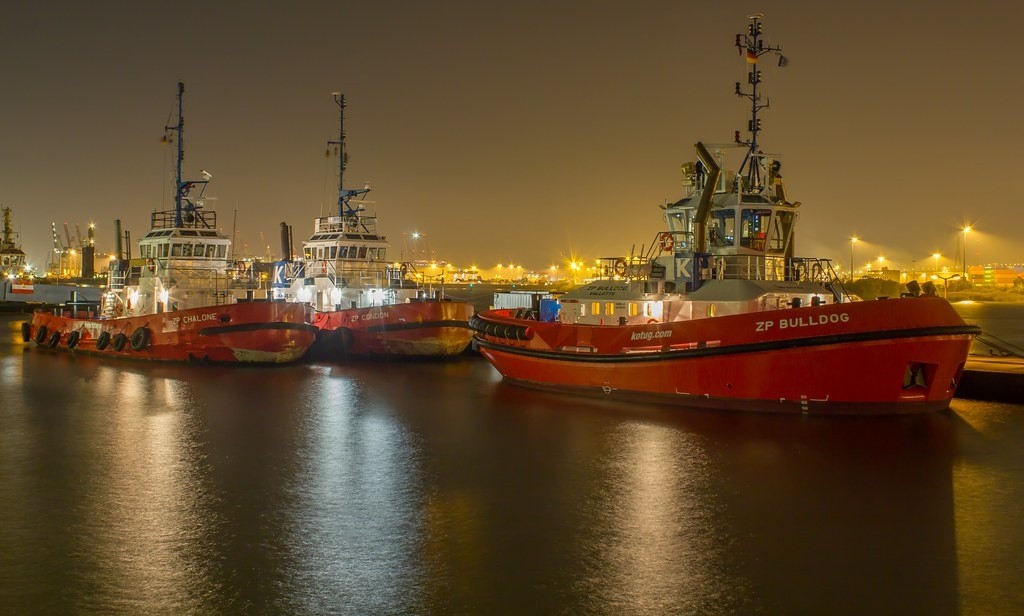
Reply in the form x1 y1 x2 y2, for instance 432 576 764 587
850 235 859 280
933 254 940 272
930 273 961 298
912 260 916 280
963 226 970 277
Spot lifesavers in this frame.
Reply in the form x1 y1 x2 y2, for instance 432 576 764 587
615 260 628 276
659 232 674 252
96 331 110 350
113 333 126 351
335 328 354 351
238 261 247 275
130 327 148 351
145 258 155 272
22 322 30 342
36 325 47 343
318 329 332 349
321 262 327 272
48 331 60 347
399 263 407 275
66 331 79 349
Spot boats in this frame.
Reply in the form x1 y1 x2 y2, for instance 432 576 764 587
468 14 983 413
20 82 476 368
